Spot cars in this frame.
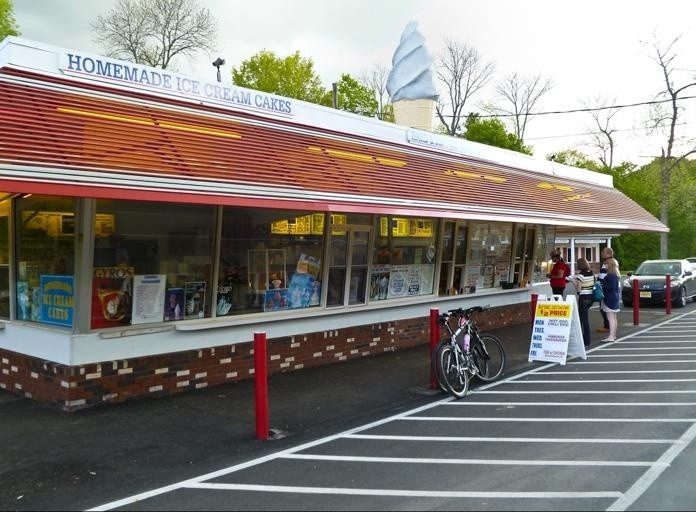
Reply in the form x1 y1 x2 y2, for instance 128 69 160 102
621 258 696 310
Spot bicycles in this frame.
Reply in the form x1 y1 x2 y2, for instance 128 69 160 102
432 304 491 396
439 306 508 402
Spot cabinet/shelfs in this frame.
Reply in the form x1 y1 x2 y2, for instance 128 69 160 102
20 237 58 287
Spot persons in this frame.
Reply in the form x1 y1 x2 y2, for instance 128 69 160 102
565 257 597 347
594 246 615 332
600 258 620 343
546 249 567 299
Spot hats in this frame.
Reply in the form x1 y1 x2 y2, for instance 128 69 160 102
550 247 561 255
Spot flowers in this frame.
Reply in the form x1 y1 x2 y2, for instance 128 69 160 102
220 259 247 284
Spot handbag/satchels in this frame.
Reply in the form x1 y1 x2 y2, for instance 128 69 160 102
593 281 605 301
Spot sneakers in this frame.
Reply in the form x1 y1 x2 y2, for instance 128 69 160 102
598 328 609 332
600 337 616 342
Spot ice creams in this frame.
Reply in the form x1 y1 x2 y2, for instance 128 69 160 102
386 18 441 132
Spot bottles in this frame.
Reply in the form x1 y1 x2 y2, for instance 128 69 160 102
174 303 181 320
463 334 470 351
